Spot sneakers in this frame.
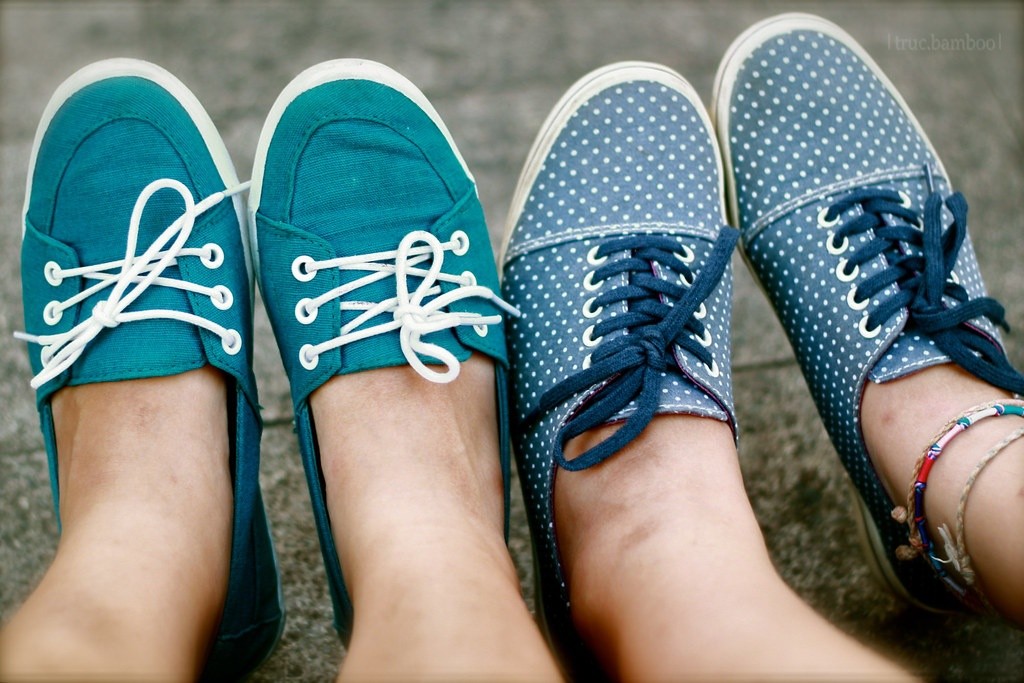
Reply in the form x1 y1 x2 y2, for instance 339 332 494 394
240 59 517 656
13 58 282 683
496 62 747 683
715 11 1024 618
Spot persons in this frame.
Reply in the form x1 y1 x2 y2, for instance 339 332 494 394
0 57 566 683
501 12 1024 683
